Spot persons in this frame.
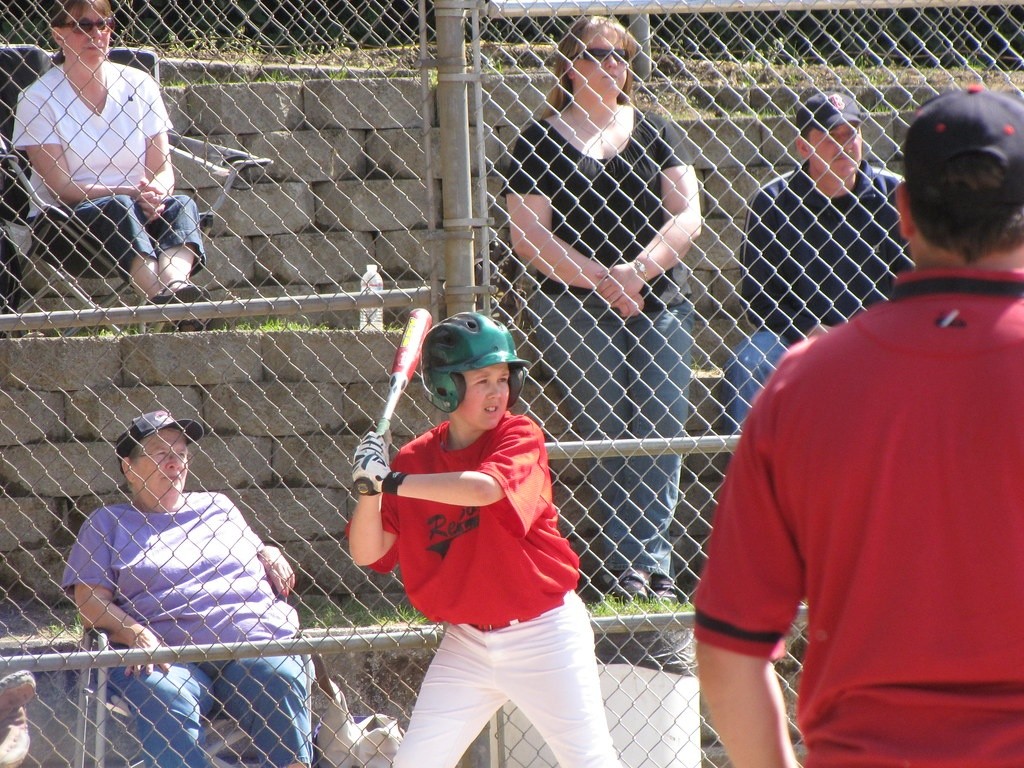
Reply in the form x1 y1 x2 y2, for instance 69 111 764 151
345 311 625 768
61 410 314 768
500 14 702 601
12 0 224 332
694 84 1024 768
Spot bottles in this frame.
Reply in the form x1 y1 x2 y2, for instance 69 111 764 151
359 264 383 331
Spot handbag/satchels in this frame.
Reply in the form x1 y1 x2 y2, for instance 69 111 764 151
314 678 405 768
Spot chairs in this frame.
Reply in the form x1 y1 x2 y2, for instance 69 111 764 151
73 626 315 767
0 44 273 333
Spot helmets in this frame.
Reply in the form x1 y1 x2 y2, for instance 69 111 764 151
421 312 530 413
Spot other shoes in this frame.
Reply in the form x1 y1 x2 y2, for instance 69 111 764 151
649 575 678 606
600 569 651 602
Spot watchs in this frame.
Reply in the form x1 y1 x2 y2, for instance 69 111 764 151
632 259 648 282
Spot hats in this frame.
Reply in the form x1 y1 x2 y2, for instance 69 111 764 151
116 410 204 460
795 91 869 135
905 85 1024 209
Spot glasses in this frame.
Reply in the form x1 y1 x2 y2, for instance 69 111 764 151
56 17 115 35
569 48 629 64
138 450 194 468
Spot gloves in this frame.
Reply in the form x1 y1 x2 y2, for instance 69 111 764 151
352 431 392 492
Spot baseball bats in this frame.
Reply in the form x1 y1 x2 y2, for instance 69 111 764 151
352 308 432 495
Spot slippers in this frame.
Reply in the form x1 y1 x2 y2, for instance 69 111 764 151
145 280 210 305
172 318 212 331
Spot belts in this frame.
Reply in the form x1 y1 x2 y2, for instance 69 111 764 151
468 623 510 632
